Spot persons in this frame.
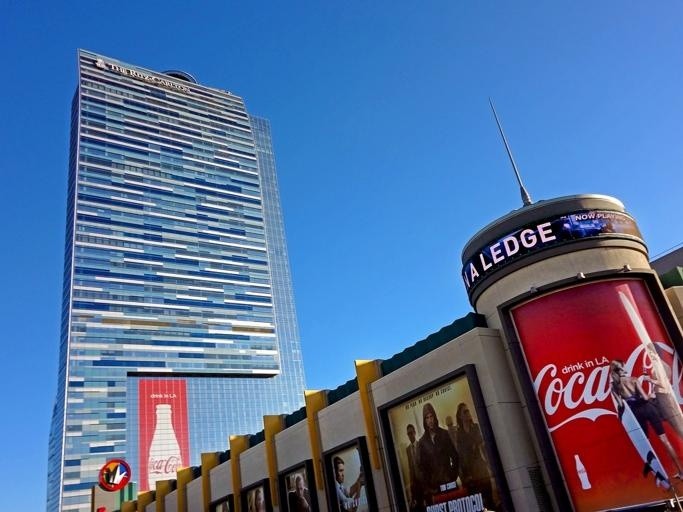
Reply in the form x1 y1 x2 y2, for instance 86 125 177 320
333 456 366 512
610 360 683 481
254 489 266 512
222 502 229 512
644 342 683 440
288 475 312 512
405 402 499 512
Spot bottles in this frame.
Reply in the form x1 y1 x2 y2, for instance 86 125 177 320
574 454 591 490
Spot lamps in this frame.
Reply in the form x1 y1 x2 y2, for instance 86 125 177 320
616 264 631 273
576 272 586 279
530 287 538 293
